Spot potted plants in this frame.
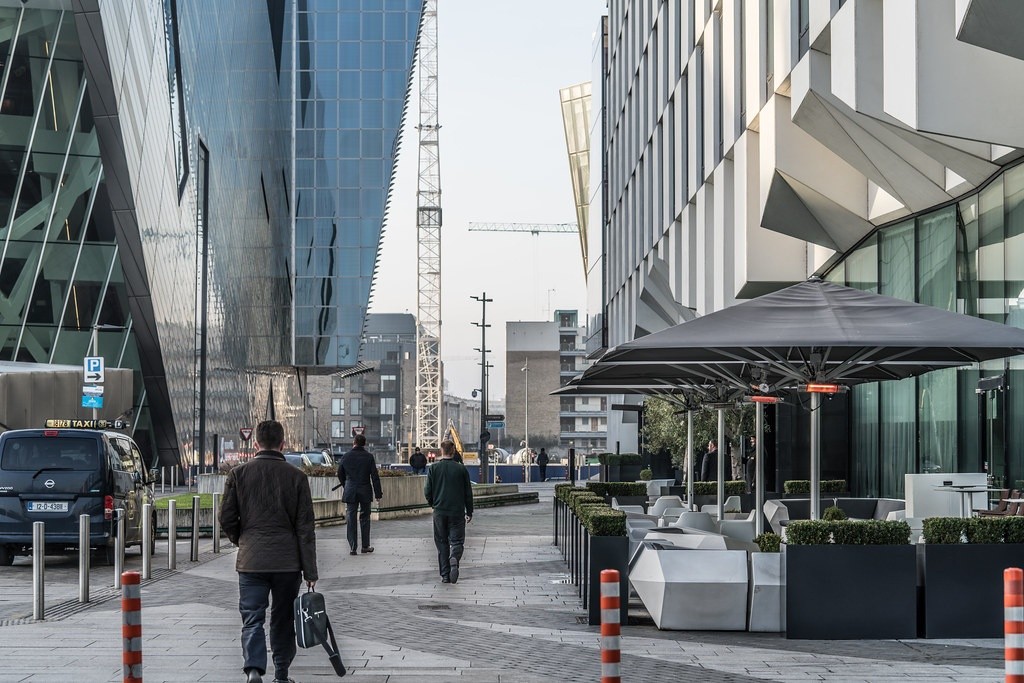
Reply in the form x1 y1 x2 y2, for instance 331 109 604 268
553 482 649 626
922 517 1023 639
636 469 676 496
723 509 749 520
598 453 642 483
779 505 917 639
683 480 851 513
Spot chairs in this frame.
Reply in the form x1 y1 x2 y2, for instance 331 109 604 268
611 495 756 541
972 491 1024 519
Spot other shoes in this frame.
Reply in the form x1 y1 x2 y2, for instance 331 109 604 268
442 577 448 583
449 557 459 584
361 546 374 553
350 550 357 554
247 669 263 683
274 677 294 683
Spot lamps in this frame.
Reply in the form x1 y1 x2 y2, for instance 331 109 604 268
704 402 736 410
743 396 784 404
797 385 846 394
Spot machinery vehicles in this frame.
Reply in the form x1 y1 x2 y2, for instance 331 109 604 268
486 443 509 464
441 418 479 465
506 446 539 465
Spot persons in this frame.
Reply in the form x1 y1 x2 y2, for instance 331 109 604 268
218 420 319 683
536 448 549 482
424 440 473 584
741 432 769 491
454 447 464 465
410 447 427 475
337 435 382 554
701 439 732 482
532 449 537 463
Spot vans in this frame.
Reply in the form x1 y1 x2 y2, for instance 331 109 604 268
0 416 162 566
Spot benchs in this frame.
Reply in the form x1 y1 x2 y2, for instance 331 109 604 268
623 519 780 632
762 497 906 537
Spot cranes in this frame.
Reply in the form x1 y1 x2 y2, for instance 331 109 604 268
467 220 581 235
414 0 444 464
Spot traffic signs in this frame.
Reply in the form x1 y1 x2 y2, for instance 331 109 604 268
485 415 505 421
486 422 505 429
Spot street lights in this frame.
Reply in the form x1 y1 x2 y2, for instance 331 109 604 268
521 356 532 484
468 291 495 484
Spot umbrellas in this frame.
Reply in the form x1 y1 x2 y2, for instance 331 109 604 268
549 385 749 512
582 275 1024 521
567 376 850 539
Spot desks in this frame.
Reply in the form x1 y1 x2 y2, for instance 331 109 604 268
931 483 1009 543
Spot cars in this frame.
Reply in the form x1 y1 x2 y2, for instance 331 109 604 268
281 447 348 471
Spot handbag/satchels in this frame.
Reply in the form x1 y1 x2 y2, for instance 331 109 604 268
294 583 328 649
